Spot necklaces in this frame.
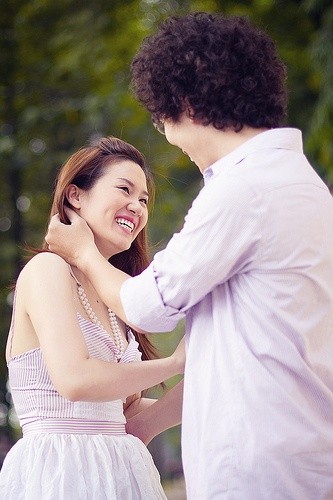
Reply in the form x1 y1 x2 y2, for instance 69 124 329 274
67 265 123 361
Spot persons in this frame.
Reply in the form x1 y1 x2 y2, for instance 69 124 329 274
0 135 187 500
43 15 333 500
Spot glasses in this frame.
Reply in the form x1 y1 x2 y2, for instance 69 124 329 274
151 99 186 135
